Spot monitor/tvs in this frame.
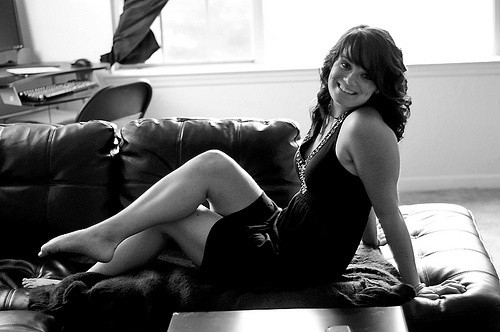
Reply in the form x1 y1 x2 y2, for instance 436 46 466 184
0 0 24 66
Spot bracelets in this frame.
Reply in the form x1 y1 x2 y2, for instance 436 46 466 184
376 235 380 244
412 281 425 292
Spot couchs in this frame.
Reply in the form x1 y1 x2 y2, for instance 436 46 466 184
0 118 500 332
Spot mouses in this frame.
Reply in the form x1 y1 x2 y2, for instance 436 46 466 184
74 59 91 67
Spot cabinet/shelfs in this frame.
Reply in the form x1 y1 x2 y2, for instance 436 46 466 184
0 57 106 120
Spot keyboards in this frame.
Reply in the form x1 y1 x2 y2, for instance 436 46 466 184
18 79 96 103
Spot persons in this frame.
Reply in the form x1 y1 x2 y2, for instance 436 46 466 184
22 25 468 301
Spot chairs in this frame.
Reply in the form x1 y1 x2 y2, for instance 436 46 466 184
75 80 153 122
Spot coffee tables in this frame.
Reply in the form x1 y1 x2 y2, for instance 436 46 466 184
168 307 407 332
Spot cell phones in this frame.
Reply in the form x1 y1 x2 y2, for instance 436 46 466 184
325 325 352 332
